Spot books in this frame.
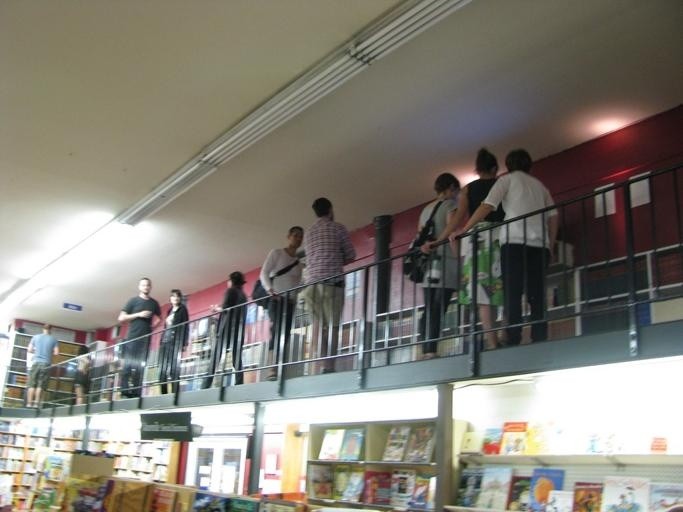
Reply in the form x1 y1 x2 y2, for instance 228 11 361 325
361 469 437 509
3 323 99 409
572 482 604 511
528 468 565 510
461 421 671 456
454 467 485 506
601 476 651 512
2 421 172 511
382 425 436 462
477 467 513 510
546 490 574 511
318 427 366 461
153 484 306 511
309 464 365 502
507 476 532 510
648 482 681 510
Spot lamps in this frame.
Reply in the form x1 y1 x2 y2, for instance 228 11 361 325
47 221 134 295
0 268 51 323
197 47 370 167
350 1 470 64
107 157 216 242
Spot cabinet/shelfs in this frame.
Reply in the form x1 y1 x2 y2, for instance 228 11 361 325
311 319 370 374
370 297 468 368
89 365 178 402
31 436 83 512
305 416 466 511
443 453 682 512
0 332 106 410
0 429 46 500
177 340 266 391
468 266 578 353
92 440 179 484
579 244 683 336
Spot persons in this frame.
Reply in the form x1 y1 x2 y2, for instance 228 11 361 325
259 226 304 381
413 172 460 359
118 277 162 398
303 197 356 374
449 148 559 346
424 146 506 350
199 271 246 389
159 288 190 393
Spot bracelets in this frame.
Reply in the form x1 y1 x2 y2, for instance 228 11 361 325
464 227 469 232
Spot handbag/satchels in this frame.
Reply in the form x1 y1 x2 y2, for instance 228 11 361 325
402 231 437 283
252 278 273 309
